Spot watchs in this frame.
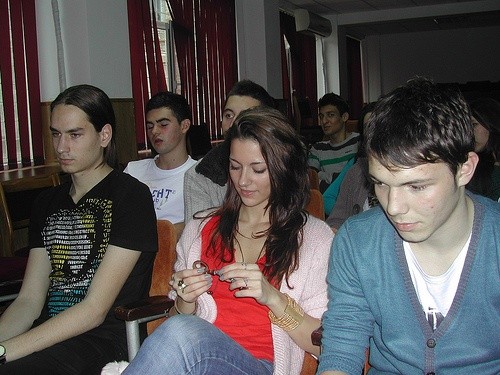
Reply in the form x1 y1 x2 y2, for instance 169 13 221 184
0 345 8 365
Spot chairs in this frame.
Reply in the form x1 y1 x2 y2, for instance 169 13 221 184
302 119 360 222
1 219 177 364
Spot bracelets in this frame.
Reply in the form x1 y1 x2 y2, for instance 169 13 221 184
175 296 198 317
268 292 306 333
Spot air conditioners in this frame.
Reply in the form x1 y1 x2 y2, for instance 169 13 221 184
294 8 332 38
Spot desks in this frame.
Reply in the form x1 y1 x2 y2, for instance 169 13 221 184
0 162 76 260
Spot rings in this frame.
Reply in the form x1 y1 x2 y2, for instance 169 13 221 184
242 263 248 270
178 279 187 293
243 277 250 288
170 274 178 285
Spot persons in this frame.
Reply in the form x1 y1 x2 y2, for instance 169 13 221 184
306 92 365 194
183 79 283 228
464 96 500 204
315 76 500 375
326 155 378 234
121 91 203 224
0 83 161 375
320 102 375 218
118 104 338 375
